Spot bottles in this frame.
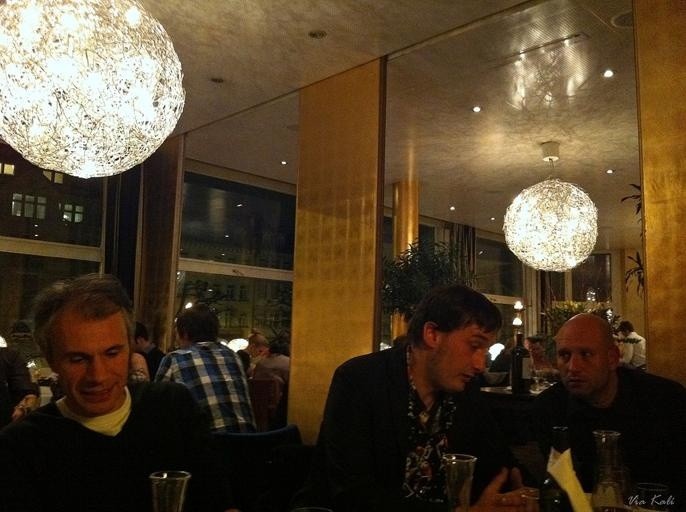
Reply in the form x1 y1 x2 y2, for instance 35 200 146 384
539 426 574 512
149 470 192 512
442 453 477 512
590 430 625 512
510 332 532 393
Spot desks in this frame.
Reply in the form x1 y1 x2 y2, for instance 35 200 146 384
479 383 563 448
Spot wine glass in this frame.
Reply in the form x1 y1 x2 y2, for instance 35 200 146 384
532 364 543 391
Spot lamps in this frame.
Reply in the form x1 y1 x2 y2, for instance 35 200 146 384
0 0 187 180
502 140 599 273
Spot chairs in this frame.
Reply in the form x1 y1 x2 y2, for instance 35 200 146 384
213 423 305 512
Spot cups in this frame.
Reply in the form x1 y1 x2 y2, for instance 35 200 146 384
631 482 669 512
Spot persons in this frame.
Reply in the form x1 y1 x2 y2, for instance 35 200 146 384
619 322 647 372
0 274 215 512
530 314 686 512
129 306 291 434
0 321 41 426
290 284 542 512
